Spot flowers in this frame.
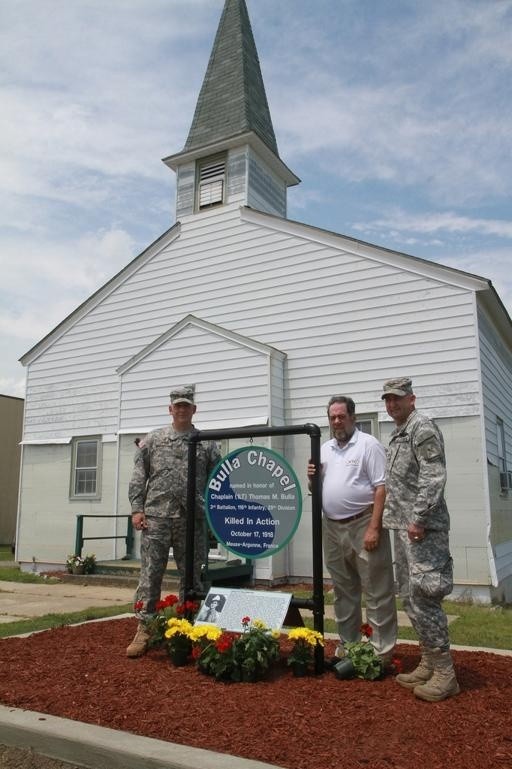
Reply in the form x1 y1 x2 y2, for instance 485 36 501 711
132 591 403 684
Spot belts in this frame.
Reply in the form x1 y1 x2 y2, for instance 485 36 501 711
327 513 362 524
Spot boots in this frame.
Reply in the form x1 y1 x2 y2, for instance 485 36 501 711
396 645 460 701
125 621 158 658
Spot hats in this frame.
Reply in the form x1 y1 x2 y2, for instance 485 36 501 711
171 387 196 406
381 377 413 400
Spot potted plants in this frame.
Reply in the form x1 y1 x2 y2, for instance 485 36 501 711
67 552 97 575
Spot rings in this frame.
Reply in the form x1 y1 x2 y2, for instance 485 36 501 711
413 536 420 540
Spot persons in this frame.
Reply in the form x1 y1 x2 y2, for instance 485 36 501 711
125 383 224 659
198 593 225 626
378 375 462 702
307 394 397 677
